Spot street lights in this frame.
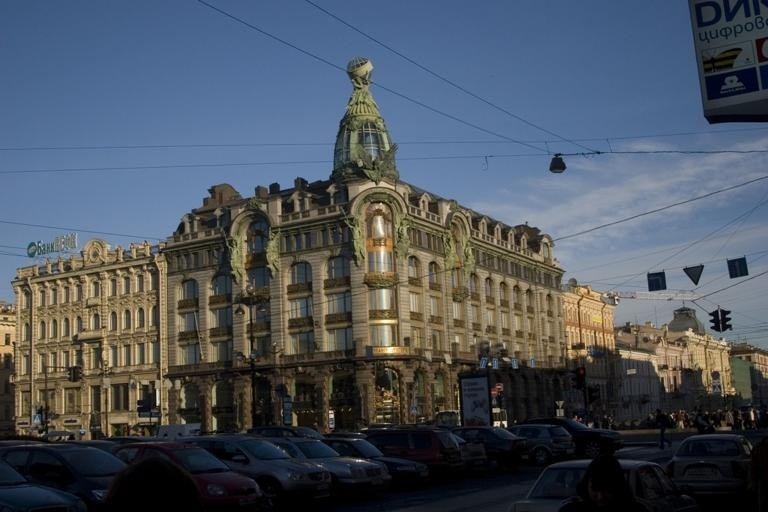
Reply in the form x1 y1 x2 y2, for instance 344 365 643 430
264 342 285 426
98 360 114 439
235 284 267 428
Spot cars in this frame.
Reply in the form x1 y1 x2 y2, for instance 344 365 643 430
508 433 768 512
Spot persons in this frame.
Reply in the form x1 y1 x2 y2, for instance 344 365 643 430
571 414 582 422
649 406 733 449
510 419 517 426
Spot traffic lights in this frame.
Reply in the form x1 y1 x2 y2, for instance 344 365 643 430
571 368 585 390
709 308 732 332
38 408 42 414
137 399 149 412
67 366 83 382
